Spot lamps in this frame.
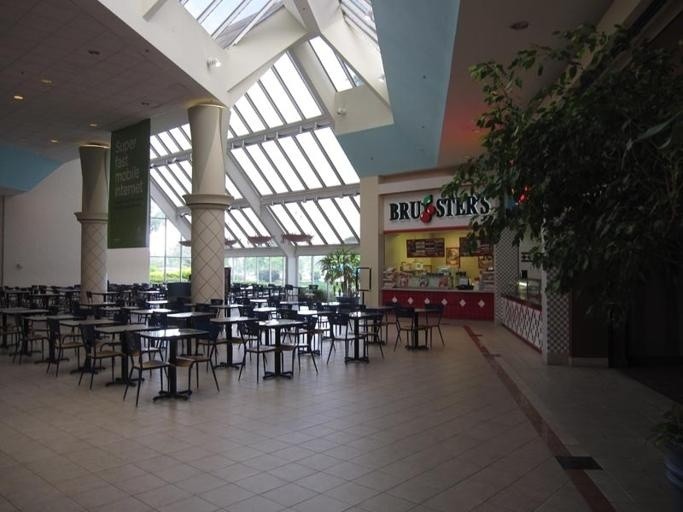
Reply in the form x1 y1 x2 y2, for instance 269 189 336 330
207 56 221 68
337 107 346 117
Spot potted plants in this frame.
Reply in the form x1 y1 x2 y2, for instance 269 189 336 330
316 252 360 315
644 399 683 488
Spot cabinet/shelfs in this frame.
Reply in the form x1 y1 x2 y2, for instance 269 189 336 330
508 276 542 305
